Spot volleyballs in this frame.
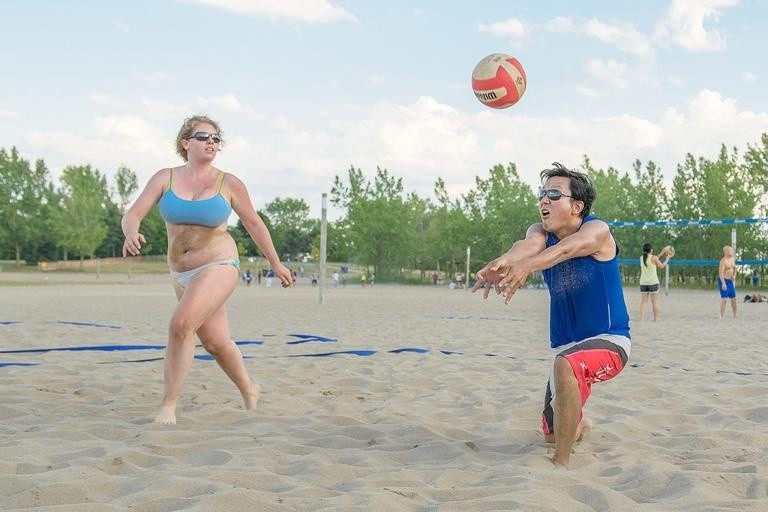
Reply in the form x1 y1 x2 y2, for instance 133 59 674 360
471 53 526 108
663 246 675 257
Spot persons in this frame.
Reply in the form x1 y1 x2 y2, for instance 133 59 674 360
457 272 464 289
472 161 632 468
638 243 671 321
752 271 759 286
120 115 295 426
745 294 768 302
243 265 376 289
717 245 739 320
432 271 442 286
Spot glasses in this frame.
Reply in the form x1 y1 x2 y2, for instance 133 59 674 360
539 189 573 200
187 132 221 143
